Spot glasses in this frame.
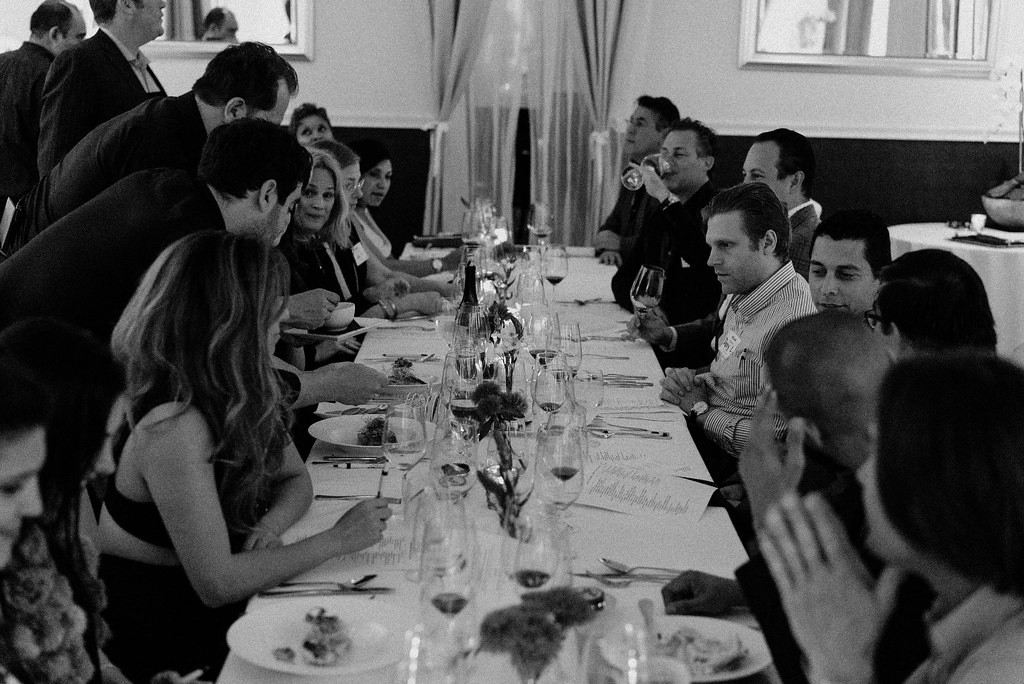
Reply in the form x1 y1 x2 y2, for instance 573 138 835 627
865 309 880 330
344 178 365 191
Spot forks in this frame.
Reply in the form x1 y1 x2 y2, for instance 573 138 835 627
314 488 424 503
376 326 435 332
556 346 630 359
586 571 670 588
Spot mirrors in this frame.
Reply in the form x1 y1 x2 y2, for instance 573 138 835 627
738 0 1004 80
84 0 316 61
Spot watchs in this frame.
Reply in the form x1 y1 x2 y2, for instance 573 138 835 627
691 401 708 421
432 258 443 273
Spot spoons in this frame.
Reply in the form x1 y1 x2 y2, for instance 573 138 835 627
575 298 602 306
277 575 378 589
598 557 684 575
590 429 672 439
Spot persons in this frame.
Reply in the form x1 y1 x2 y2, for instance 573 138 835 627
593 94 1024 684
0 0 168 224
201 6 238 43
0 41 470 684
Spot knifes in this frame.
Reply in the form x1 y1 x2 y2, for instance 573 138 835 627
258 588 395 600
574 574 679 582
324 456 430 462
555 336 624 340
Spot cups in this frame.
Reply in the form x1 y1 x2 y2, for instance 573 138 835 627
970 214 986 232
324 302 355 331
575 370 604 412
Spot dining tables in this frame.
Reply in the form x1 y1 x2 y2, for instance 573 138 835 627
217 242 783 684
887 222 1024 367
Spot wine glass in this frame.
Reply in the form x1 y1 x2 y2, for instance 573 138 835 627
414 198 588 684
620 264 665 345
383 411 426 531
621 153 672 191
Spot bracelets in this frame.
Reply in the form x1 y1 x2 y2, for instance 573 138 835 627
378 300 394 322
385 297 398 316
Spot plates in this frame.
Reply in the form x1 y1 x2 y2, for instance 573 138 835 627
308 414 442 456
381 375 443 397
226 601 411 676
596 616 774 682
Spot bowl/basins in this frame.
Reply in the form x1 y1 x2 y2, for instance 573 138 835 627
980 195 1024 228
